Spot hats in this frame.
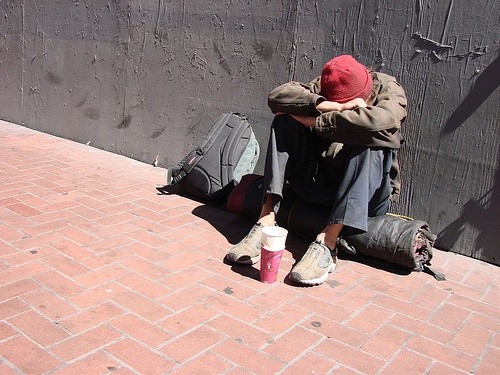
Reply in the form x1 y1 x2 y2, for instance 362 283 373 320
320 55 373 103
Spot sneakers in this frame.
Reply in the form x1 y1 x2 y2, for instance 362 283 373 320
288 241 339 285
224 220 279 265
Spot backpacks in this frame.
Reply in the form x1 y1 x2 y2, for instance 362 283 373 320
166 111 260 210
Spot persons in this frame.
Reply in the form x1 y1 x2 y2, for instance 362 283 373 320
228 55 408 285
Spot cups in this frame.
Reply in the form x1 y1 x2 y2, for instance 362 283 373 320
260 247 285 283
261 226 288 249
